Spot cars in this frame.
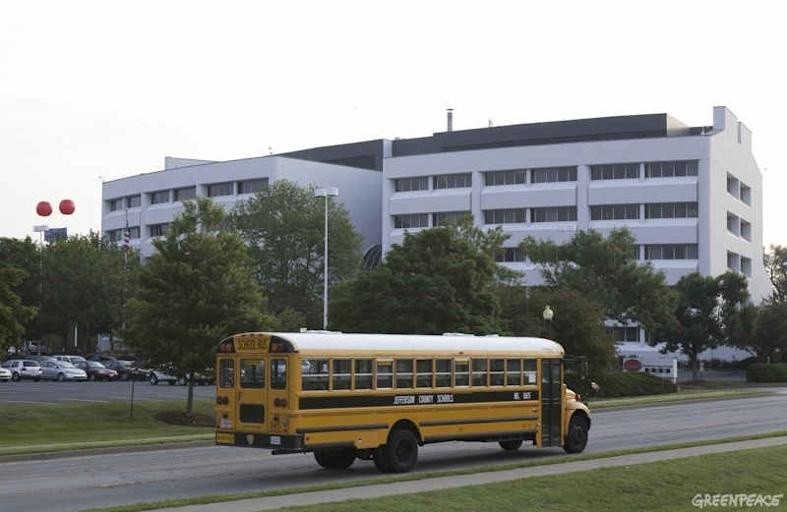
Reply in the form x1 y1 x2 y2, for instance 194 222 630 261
147 362 247 385
0 352 154 382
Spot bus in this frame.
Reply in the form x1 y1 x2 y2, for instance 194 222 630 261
212 331 590 472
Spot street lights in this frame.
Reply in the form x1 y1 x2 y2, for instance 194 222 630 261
314 187 340 329
543 305 554 338
33 225 49 293
